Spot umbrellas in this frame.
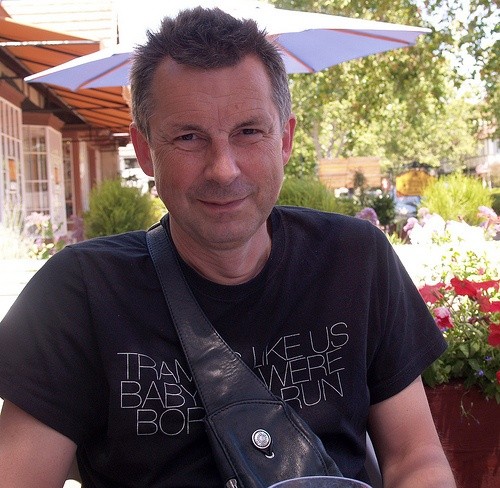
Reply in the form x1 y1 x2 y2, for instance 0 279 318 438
24 4 432 92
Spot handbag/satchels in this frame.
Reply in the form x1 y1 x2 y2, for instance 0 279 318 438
147 222 383 488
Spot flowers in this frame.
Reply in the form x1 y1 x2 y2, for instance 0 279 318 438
413 224 500 408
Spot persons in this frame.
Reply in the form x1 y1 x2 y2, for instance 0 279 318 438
0 5 458 488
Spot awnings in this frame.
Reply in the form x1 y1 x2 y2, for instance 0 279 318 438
0 17 136 133
